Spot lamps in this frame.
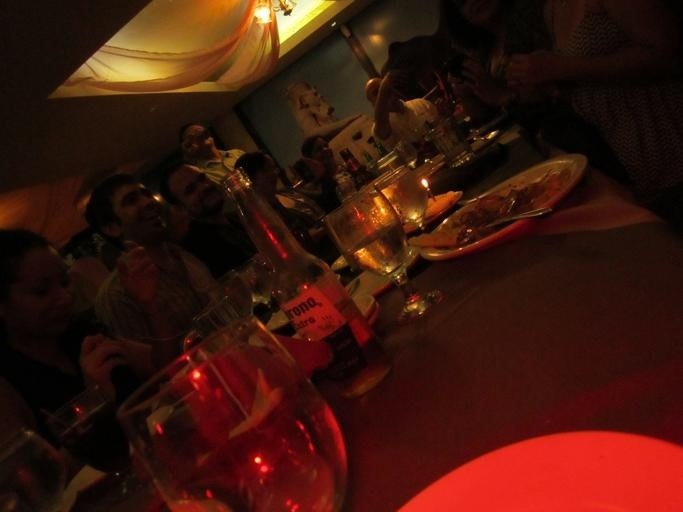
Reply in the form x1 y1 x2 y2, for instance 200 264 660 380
253 0 296 24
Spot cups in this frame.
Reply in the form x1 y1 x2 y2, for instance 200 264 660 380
317 98 496 174
0 379 145 511
178 272 256 340
116 318 348 512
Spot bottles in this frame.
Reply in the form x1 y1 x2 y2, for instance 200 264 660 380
221 169 394 399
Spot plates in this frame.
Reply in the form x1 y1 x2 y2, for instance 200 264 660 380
396 428 683 512
423 151 589 262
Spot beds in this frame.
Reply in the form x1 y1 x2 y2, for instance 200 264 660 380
318 122 683 512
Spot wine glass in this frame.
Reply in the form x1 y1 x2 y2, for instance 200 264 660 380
324 190 447 326
94 230 140 275
375 166 436 244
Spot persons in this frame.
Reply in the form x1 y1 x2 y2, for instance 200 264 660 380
364 0 682 232
1 120 344 497
